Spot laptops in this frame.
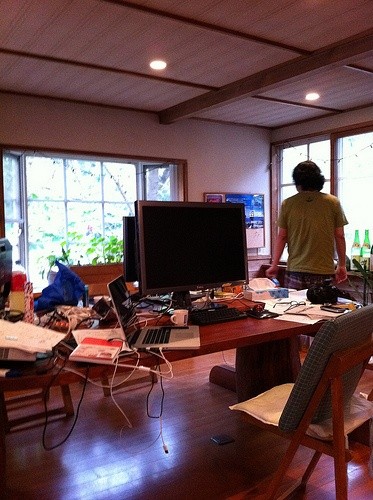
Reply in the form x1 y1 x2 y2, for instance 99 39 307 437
107 274 201 351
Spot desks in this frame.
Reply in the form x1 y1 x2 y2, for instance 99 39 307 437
262 264 373 306
0 294 363 393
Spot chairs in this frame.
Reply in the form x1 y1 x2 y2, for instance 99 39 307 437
0 384 74 436
228 304 373 500
86 282 159 398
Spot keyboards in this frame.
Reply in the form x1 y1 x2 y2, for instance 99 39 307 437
187 306 248 325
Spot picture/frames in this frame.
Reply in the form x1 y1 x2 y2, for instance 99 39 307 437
203 192 226 203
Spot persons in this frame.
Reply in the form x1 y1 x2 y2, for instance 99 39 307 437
265 160 349 290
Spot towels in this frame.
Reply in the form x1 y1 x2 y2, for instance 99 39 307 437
33 261 86 312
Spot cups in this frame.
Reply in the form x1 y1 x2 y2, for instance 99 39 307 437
170 309 188 326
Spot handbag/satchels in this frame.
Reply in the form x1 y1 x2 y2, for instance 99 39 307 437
33 261 86 311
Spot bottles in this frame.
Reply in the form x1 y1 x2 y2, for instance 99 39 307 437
12 260 25 276
351 230 361 270
361 230 373 272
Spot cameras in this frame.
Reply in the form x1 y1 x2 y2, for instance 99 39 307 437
307 278 339 305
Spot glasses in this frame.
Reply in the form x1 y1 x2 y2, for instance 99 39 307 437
273 301 306 312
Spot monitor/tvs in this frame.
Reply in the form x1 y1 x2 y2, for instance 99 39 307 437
123 215 181 302
135 200 248 312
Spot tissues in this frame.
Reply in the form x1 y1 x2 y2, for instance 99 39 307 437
243 277 289 301
